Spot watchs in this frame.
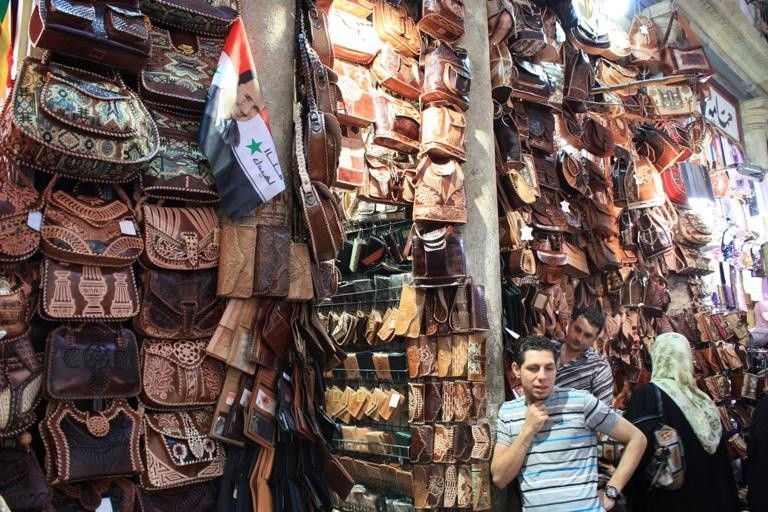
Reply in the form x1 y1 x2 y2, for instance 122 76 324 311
602 485 620 501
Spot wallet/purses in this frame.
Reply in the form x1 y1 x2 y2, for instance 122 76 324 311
326 384 343 422
418 333 439 377
332 386 356 425
470 462 491 511
207 297 352 512
338 274 405 301
340 425 410 459
472 382 486 418
342 353 360 380
428 464 445 508
443 463 458 508
388 352 408 384
408 382 425 425
339 224 405 276
365 387 387 422
346 386 371 422
413 463 431 510
451 334 468 378
411 424 432 465
471 422 493 463
216 223 258 299
356 351 375 379
453 424 472 463
341 455 413 511
405 338 421 379
253 224 291 299
318 306 396 345
454 379 474 422
468 335 487 382
395 284 426 339
372 353 391 383
289 242 314 303
441 380 455 424
379 388 405 423
436 336 452 378
433 423 454 464
457 463 472 509
424 381 442 423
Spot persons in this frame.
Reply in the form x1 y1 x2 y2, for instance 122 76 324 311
553 305 614 408
218 70 263 147
489 336 648 512
619 332 740 512
737 372 768 512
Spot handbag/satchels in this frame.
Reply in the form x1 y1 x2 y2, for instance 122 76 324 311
333 0 376 20
412 155 469 225
27 0 153 77
420 0 466 42
0 48 160 185
292 102 346 264
493 126 768 505
37 397 145 487
139 105 222 204
0 338 44 440
44 322 142 400
488 0 716 126
0 261 39 345
40 170 144 268
333 58 376 129
363 144 416 207
334 131 367 191
426 284 490 339
403 221 466 290
136 25 228 121
343 185 406 225
307 1 336 71
370 42 424 101
53 475 136 512
298 34 342 188
0 147 44 263
136 194 220 273
372 0 421 59
136 337 227 412
134 484 216 512
36 256 140 324
142 0 240 38
418 100 469 162
0 443 53 512
136 404 226 490
372 89 421 155
327 5 378 65
133 266 228 340
310 259 342 301
420 39 472 112
298 7 346 116
